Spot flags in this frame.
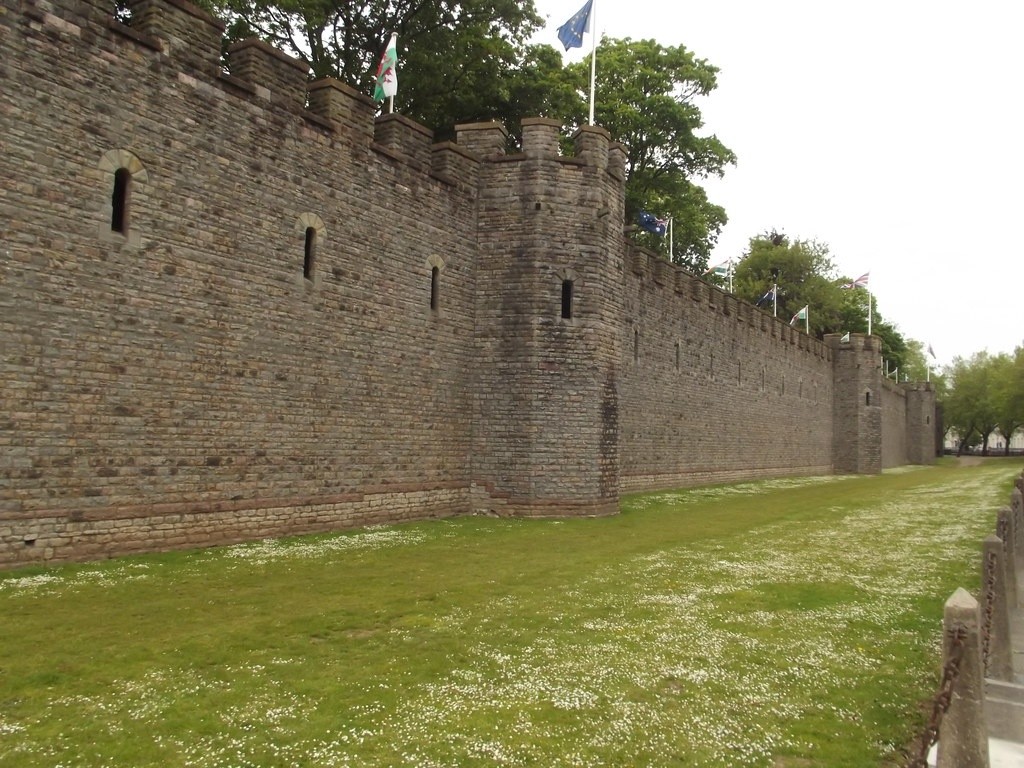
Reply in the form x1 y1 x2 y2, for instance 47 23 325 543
755 286 775 306
640 211 671 236
790 307 806 325
841 273 870 289
557 0 593 51
928 344 936 359
841 334 849 343
701 260 729 275
374 38 397 102
